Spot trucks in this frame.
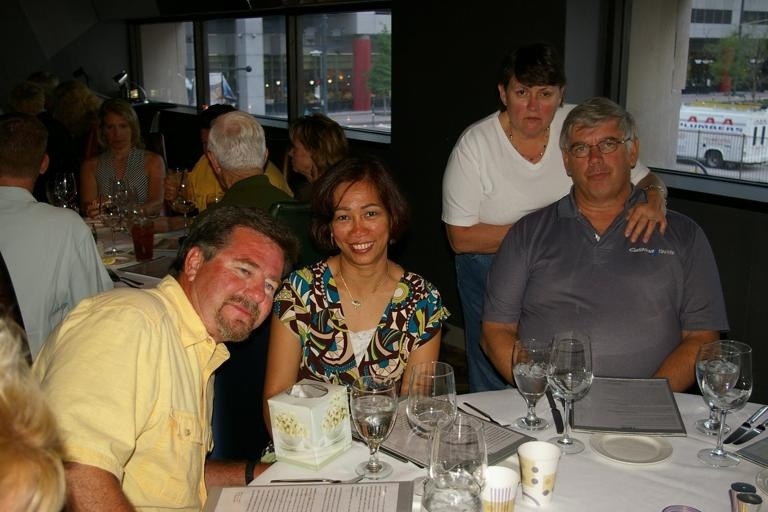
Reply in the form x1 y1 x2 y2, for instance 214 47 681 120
673 94 767 169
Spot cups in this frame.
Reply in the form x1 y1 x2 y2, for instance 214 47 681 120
472 466 518 512
96 239 115 264
131 219 153 264
405 360 487 511
517 441 561 507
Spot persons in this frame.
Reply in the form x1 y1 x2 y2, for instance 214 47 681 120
1 72 349 512
441 40 668 393
477 97 731 393
262 157 452 447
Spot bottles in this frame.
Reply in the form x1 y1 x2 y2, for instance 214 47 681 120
728 481 763 512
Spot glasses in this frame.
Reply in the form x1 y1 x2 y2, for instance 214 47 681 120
570 139 631 158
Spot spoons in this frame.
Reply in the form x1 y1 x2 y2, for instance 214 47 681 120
107 268 144 289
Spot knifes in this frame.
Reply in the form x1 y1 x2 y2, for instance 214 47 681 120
541 381 564 436
720 405 767 445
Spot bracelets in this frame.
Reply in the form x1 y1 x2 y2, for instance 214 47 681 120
640 184 667 205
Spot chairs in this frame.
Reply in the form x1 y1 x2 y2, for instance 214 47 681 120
145 132 167 168
266 198 314 241
207 339 270 464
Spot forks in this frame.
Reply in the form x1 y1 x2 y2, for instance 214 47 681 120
270 474 367 484
462 401 511 427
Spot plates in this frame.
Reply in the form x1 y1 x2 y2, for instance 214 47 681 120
755 468 768 496
589 431 673 464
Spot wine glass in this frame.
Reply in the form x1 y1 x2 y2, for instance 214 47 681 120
693 338 755 472
47 166 217 256
508 337 552 434
548 329 595 457
350 373 398 479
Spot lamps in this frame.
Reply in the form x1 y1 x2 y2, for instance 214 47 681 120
110 68 151 104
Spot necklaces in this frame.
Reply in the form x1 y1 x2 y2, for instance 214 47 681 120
338 259 391 311
509 121 549 163
